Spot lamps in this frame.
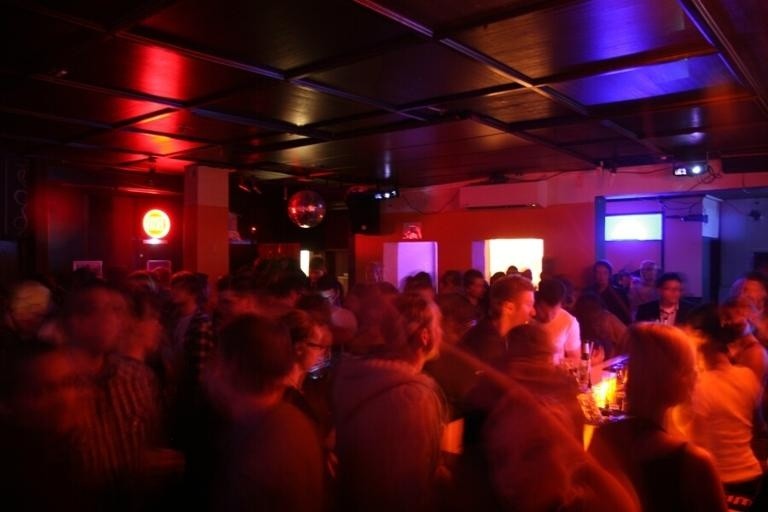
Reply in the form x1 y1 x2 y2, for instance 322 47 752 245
374 190 399 200
287 190 327 228
